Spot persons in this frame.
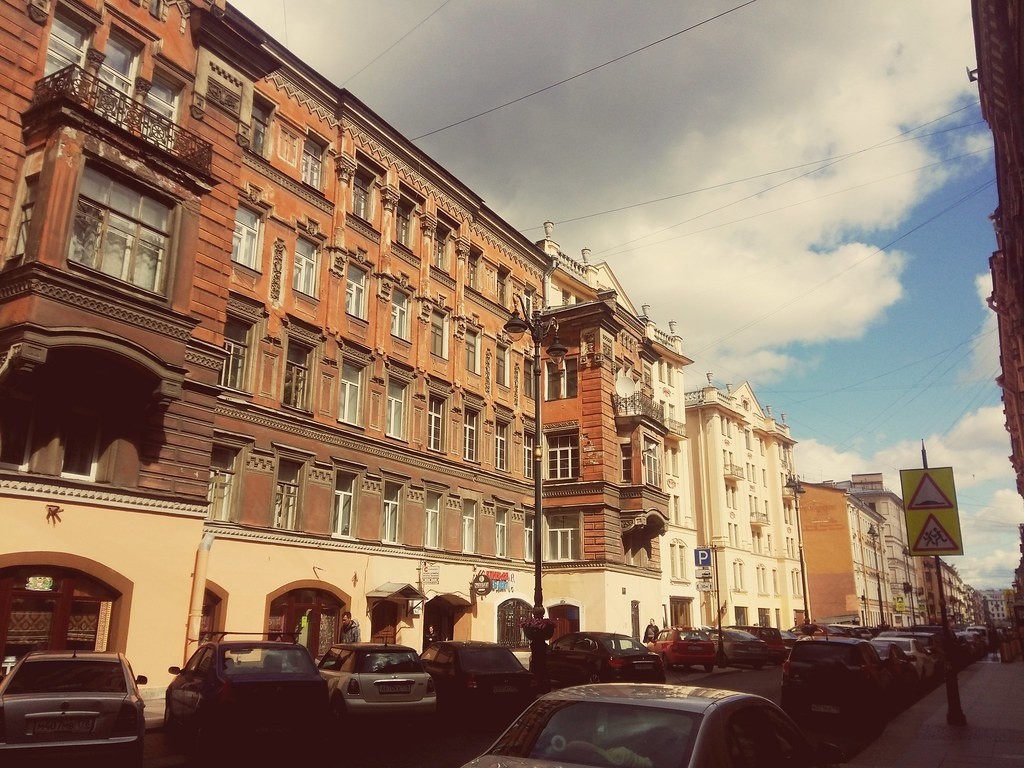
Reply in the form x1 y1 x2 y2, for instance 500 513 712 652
262 634 283 672
340 611 361 645
973 623 1010 659
643 619 660 645
426 625 439 648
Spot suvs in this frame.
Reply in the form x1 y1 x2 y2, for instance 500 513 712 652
729 626 785 664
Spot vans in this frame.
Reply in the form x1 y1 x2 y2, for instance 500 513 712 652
965 626 990 650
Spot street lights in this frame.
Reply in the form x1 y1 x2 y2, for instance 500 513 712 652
867 522 886 628
947 577 957 625
503 292 568 693
902 547 916 625
785 474 813 635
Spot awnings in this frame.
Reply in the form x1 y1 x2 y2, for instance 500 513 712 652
366 582 429 600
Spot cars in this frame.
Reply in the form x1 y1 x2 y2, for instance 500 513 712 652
870 640 919 694
779 634 887 716
881 631 948 676
647 629 716 673
315 643 437 723
788 623 846 636
545 631 667 687
955 630 987 664
162 640 330 748
871 626 912 633
687 627 768 670
458 681 843 768
871 636 936 682
910 624 967 671
0 648 148 768
828 625 872 641
418 642 537 713
780 629 797 652
